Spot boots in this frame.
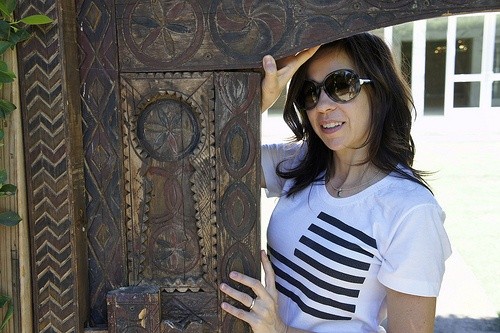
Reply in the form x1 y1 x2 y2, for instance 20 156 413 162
348 92 355 98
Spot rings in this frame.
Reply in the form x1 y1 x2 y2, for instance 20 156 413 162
249 298 256 310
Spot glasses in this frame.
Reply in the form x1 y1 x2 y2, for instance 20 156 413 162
292 67 372 112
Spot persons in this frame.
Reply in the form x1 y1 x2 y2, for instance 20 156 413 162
219 31 453 333
